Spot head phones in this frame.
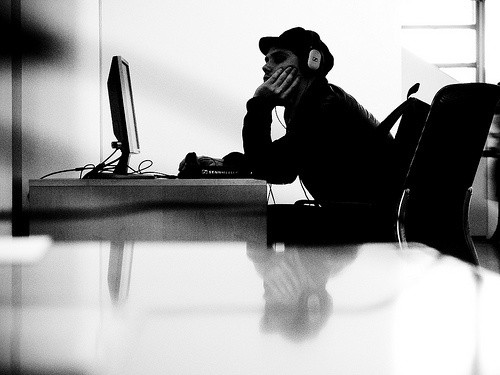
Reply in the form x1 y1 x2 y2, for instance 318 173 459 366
300 44 325 79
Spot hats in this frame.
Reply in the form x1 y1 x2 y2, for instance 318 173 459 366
259 26 334 79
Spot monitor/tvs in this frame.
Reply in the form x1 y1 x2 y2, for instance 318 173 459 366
106 56 142 155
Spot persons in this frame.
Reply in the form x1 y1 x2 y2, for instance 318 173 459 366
177 25 410 249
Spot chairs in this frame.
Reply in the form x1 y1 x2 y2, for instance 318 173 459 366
395 80 500 272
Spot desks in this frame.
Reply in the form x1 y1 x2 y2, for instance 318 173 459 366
27 179 272 250
